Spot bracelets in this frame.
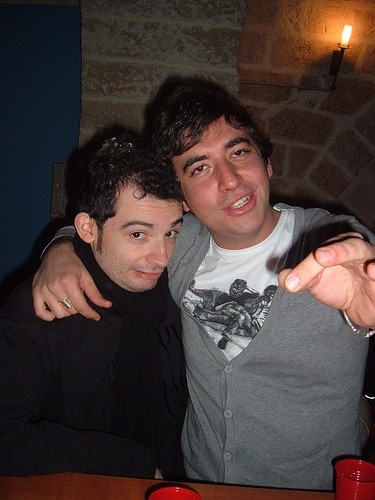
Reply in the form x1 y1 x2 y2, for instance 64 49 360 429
341 309 375 338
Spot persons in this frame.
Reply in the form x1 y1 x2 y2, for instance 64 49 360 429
33 75 375 492
0 127 190 479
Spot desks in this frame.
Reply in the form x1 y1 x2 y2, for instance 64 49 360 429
0 471 336 500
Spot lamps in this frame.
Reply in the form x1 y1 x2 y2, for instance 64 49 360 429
328 25 353 91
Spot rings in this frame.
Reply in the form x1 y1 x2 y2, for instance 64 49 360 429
59 298 72 310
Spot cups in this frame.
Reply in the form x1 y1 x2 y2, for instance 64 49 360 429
148 487 205 500
335 459 375 500
